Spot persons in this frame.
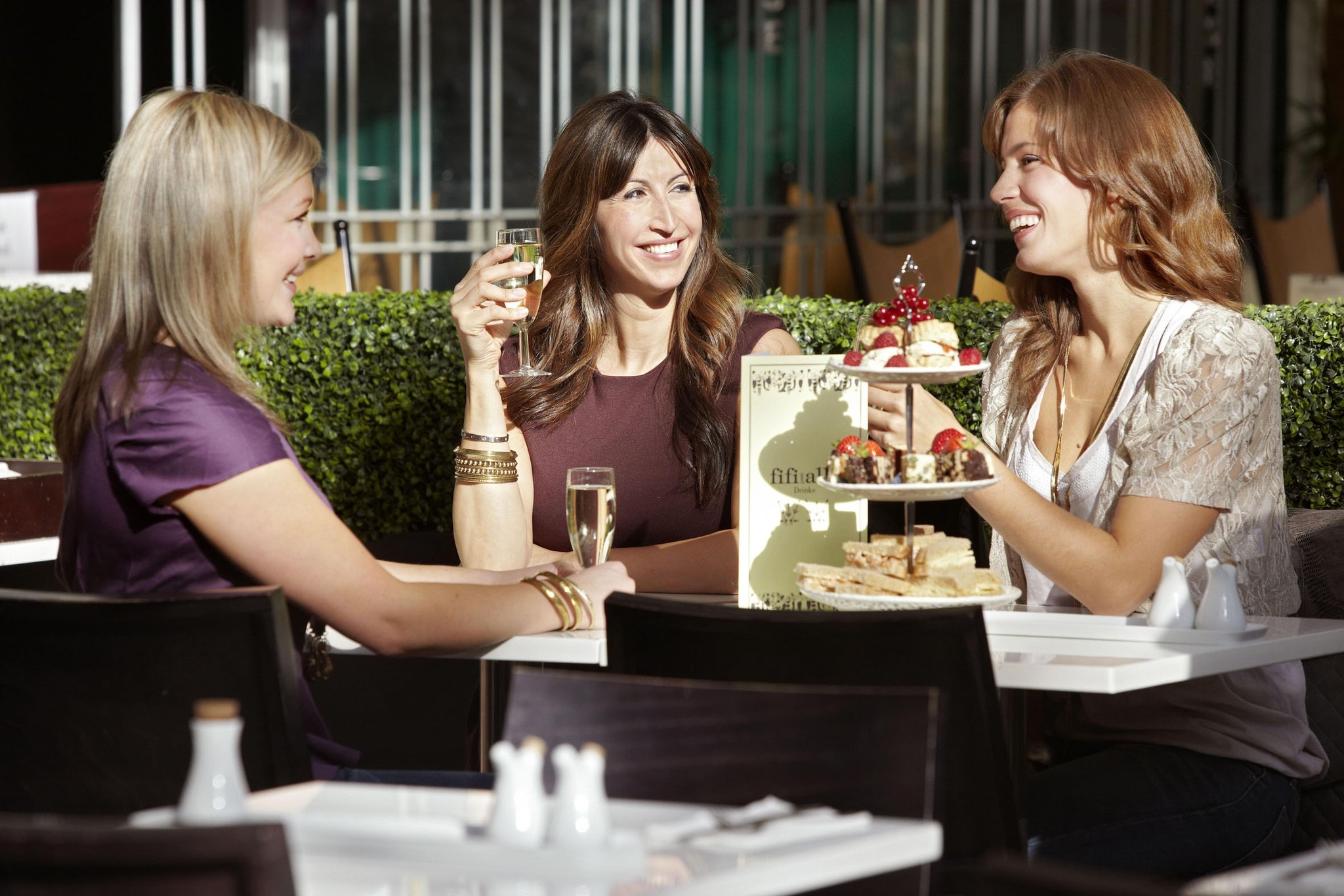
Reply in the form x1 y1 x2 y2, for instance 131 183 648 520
449 90 804 592
51 89 634 785
872 47 1332 876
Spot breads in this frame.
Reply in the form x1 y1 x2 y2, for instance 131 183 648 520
793 523 1005 597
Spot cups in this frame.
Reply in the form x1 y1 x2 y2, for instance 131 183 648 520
566 466 617 571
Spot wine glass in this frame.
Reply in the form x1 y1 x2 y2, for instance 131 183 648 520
496 228 551 377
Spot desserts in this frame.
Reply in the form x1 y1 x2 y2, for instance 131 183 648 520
842 315 962 368
825 430 988 484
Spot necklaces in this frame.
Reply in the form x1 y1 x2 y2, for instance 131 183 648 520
1051 295 1164 512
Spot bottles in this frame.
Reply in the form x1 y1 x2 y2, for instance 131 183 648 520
174 698 254 825
548 743 608 839
1196 557 1247 632
490 735 548 851
1146 555 1195 628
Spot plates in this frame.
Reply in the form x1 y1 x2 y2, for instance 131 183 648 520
985 613 1269 643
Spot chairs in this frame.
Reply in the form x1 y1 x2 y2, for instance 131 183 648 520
0 508 1343 896
1236 168 1343 305
835 196 968 305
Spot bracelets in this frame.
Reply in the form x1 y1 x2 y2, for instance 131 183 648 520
520 571 595 633
453 429 519 483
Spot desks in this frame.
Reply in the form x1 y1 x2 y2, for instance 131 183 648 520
303 606 1344 695
